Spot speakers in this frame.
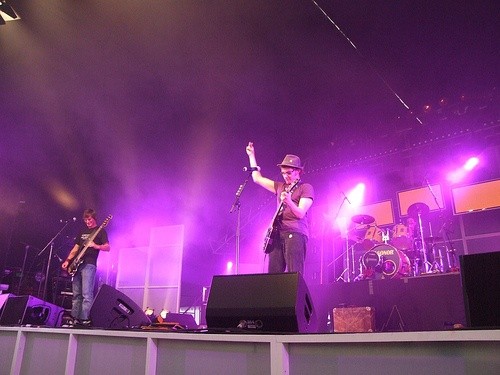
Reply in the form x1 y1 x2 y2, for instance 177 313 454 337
88 283 152 331
166 313 197 329
0 295 64 328
212 273 316 332
459 251 500 330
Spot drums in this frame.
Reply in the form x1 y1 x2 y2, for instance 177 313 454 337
391 222 415 251
362 225 385 250
364 243 411 280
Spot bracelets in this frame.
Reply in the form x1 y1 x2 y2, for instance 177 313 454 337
65 259 70 262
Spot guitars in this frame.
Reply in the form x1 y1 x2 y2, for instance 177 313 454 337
66 214 113 276
263 177 302 254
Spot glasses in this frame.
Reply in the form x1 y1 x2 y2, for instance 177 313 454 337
280 168 296 175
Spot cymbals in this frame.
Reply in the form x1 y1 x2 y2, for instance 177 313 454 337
351 214 375 224
407 202 430 219
425 236 443 242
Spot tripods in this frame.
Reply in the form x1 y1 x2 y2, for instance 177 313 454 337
336 221 364 282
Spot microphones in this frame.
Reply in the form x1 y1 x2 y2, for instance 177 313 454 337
243 166 260 171
60 217 77 222
342 192 350 204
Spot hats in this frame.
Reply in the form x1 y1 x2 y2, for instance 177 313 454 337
277 155 304 170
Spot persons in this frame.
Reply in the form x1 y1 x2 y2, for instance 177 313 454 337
62 208 109 325
245 141 315 274
